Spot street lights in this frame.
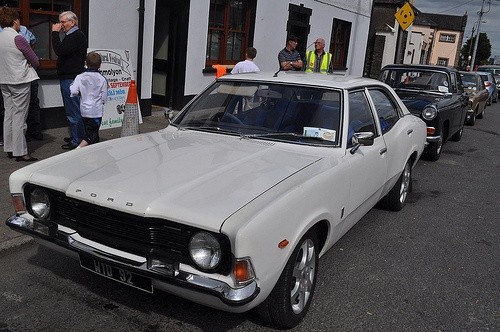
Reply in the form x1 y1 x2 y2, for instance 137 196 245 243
467 20 487 65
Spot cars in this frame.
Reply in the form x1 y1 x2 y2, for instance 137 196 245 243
463 71 498 106
4 70 427 330
437 71 489 126
351 63 470 161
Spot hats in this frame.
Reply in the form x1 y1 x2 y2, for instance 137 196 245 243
287 35 299 41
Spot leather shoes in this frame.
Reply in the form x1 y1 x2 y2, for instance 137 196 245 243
62 143 77 150
64 138 71 142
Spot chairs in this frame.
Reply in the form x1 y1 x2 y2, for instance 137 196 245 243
238 98 396 145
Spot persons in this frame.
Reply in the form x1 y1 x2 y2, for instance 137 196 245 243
69 53 108 151
0 23 42 140
0 7 40 162
303 38 333 74
278 35 304 71
51 11 87 149
230 47 260 112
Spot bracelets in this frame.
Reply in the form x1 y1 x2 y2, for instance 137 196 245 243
290 62 293 65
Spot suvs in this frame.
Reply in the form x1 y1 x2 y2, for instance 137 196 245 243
476 64 500 92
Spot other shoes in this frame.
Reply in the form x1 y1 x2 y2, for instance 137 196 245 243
33 135 43 140
26 136 31 142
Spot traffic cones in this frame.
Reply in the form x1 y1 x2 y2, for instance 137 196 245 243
120 79 140 137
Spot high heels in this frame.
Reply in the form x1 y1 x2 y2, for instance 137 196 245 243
16 155 37 162
7 152 13 159
